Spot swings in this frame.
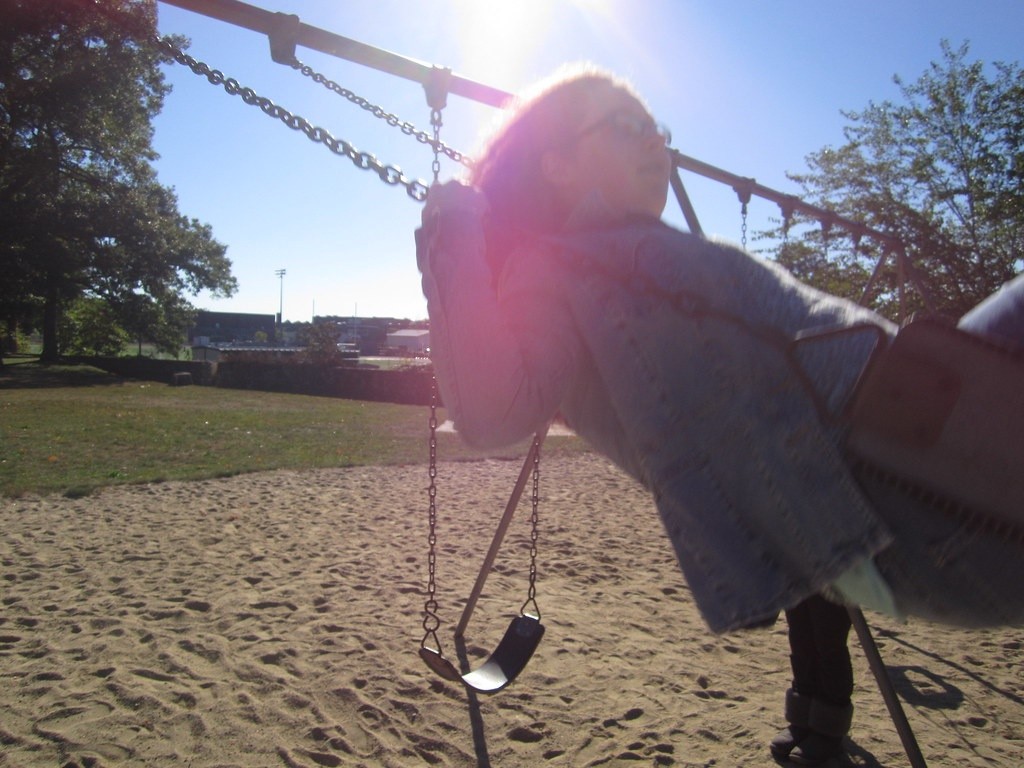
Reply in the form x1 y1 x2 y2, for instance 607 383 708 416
93 0 1023 559
417 78 549 695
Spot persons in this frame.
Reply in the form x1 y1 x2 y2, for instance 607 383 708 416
410 73 1022 763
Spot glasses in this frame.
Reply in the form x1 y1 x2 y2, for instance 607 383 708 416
557 110 670 156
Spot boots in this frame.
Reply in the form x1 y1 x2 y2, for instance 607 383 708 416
789 699 854 763
770 689 812 756
